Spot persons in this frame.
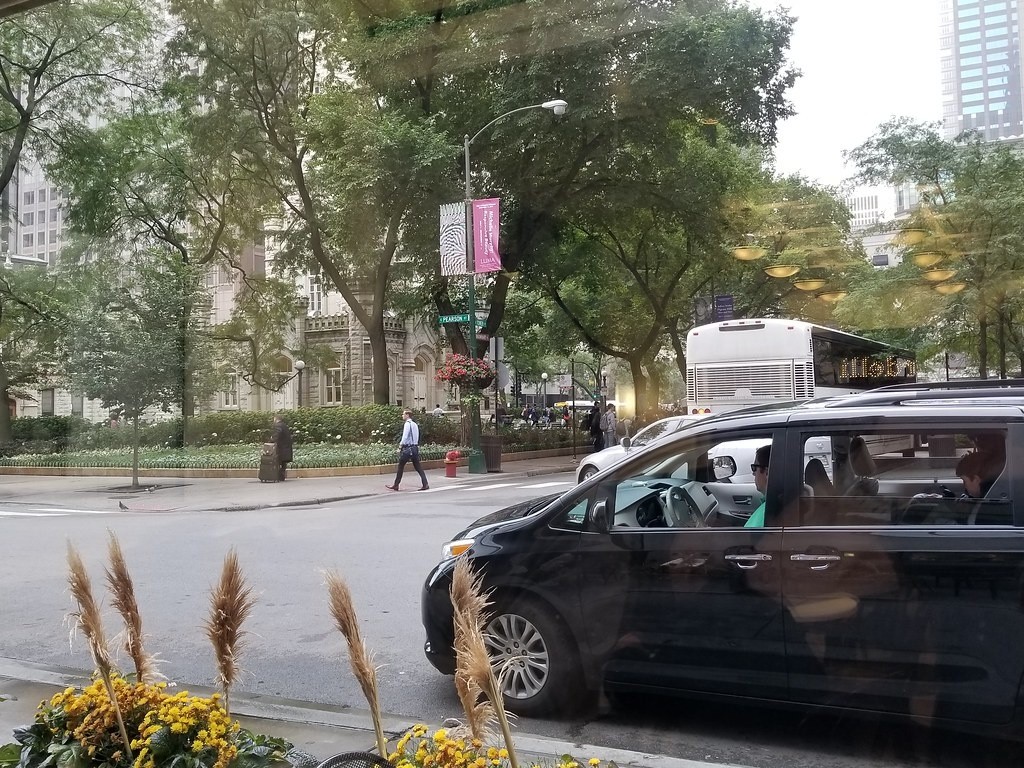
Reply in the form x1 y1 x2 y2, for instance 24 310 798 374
898 435 1004 526
488 403 572 428
421 404 443 416
587 401 616 452
744 444 771 528
386 410 429 491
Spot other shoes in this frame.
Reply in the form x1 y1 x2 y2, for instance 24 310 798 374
418 484 429 490
385 484 399 491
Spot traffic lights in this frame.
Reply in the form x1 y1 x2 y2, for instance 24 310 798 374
474 318 486 327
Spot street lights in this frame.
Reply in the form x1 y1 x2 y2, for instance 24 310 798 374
468 98 568 473
295 361 305 409
601 371 607 412
541 373 548 409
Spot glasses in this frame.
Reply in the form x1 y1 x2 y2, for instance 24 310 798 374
750 464 767 473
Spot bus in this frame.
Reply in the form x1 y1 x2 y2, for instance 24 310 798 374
686 319 916 422
553 400 615 413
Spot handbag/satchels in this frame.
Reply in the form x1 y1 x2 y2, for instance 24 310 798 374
399 446 419 460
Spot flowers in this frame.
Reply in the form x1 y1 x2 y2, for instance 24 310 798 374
433 352 499 391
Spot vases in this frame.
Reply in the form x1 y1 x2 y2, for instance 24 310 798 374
475 376 494 389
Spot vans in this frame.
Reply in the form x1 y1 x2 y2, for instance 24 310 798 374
421 386 1024 743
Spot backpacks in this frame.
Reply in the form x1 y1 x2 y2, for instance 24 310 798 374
600 412 613 430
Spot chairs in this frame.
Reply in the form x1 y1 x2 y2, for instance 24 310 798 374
314 751 397 768
800 437 882 528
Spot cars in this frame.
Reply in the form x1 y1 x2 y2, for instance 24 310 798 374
575 413 837 485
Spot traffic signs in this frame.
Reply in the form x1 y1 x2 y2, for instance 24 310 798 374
439 314 469 323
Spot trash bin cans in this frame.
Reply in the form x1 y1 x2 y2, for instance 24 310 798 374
928 436 956 468
480 435 505 473
